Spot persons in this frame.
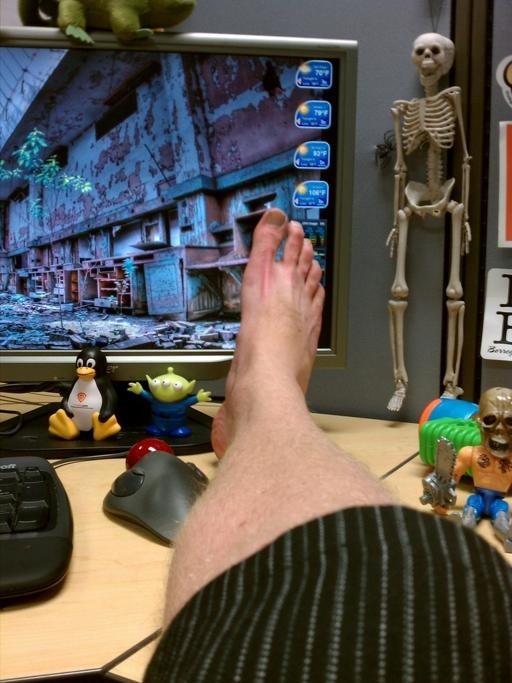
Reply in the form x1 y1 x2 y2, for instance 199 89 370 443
127 206 512 683
410 384 512 555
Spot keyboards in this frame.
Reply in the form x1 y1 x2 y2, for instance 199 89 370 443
1 454 73 599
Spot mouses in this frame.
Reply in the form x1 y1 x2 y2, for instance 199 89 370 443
103 435 210 544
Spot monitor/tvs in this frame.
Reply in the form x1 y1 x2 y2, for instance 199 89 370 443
0 25 359 454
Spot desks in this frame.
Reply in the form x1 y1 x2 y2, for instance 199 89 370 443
0 380 512 683
